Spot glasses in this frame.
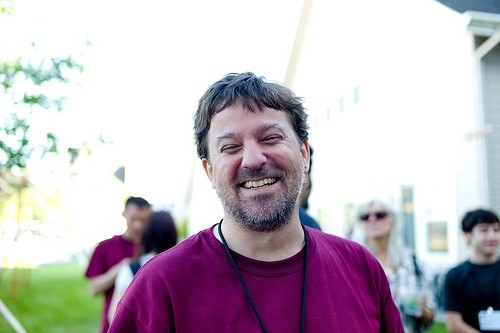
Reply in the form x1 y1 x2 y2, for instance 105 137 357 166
361 211 387 220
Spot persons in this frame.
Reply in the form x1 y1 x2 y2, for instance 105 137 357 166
107 72 405 333
85 196 154 333
108 211 179 328
440 209 500 333
356 200 436 333
299 146 322 232
